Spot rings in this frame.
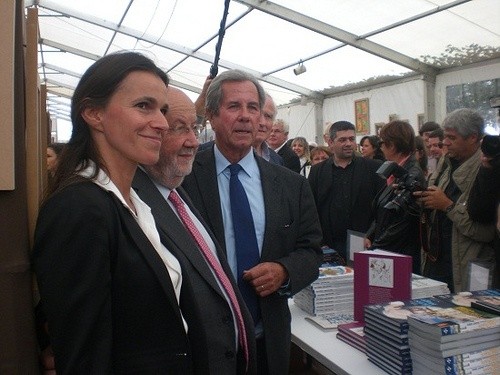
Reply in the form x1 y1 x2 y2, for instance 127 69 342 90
260 285 265 290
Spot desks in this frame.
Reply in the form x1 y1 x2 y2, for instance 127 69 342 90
288 297 396 375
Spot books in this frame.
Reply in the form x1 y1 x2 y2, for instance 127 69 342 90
292 248 500 375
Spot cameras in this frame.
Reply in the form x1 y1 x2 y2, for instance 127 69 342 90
376 161 428 218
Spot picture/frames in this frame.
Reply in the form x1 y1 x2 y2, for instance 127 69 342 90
375 122 385 138
354 97 370 136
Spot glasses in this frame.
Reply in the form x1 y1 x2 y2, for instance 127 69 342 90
169 120 205 136
376 141 386 148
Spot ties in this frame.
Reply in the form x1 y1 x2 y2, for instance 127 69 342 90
222 163 263 331
166 191 249 372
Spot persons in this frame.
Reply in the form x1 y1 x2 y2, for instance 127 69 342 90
32 49 311 375
308 108 500 294
